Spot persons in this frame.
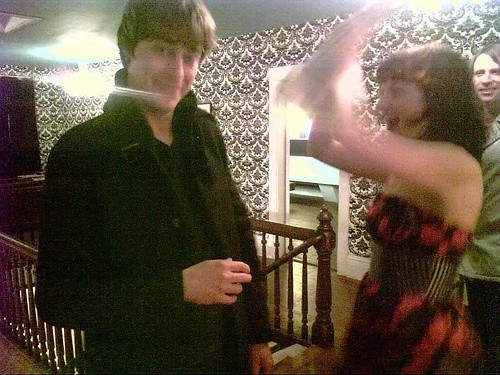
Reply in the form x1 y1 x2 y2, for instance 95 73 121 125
34 0 275 375
456 42 499 375
277 0 488 373
